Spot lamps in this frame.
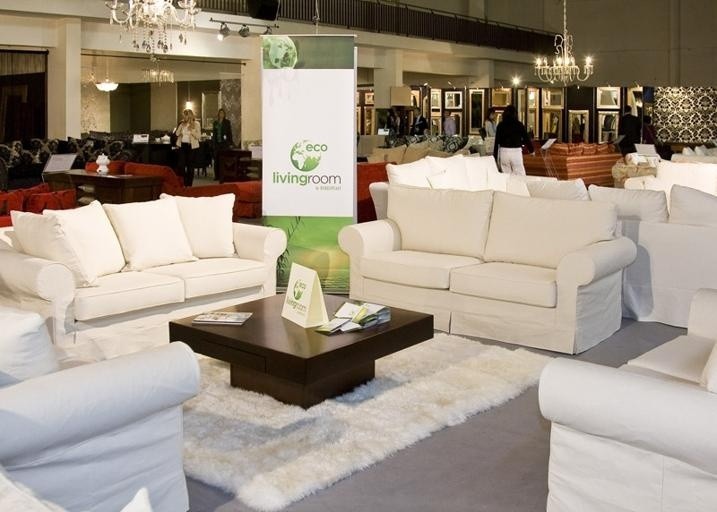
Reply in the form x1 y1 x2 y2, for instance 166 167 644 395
95 57 118 93
141 57 175 86
535 0 594 84
208 17 279 42
103 1 200 60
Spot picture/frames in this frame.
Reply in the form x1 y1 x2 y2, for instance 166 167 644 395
355 85 644 148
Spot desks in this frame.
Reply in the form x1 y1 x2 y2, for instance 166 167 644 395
170 292 434 408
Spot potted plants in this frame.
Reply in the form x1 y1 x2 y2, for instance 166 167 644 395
355 85 644 148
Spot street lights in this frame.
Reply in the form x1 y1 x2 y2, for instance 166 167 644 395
171 134 177 147
485 138 495 153
479 128 486 140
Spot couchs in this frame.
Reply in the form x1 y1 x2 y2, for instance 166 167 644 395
2 310 202 509
356 132 717 328
338 186 638 355
2 192 287 364
536 286 715 510
2 129 265 222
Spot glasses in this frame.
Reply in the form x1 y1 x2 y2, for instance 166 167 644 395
141 57 175 86
103 1 200 60
208 17 279 42
535 0 594 84
95 57 118 93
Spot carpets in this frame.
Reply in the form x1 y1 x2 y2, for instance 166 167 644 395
73 314 553 512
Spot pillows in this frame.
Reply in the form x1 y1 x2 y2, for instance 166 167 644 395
10 210 99 288
159 194 237 259
99 196 198 272
1 310 58 383
43 200 126 278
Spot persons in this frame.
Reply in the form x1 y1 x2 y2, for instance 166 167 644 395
211 109 233 181
643 116 657 144
619 106 642 157
170 120 183 153
175 109 201 188
384 105 496 137
494 106 535 175
